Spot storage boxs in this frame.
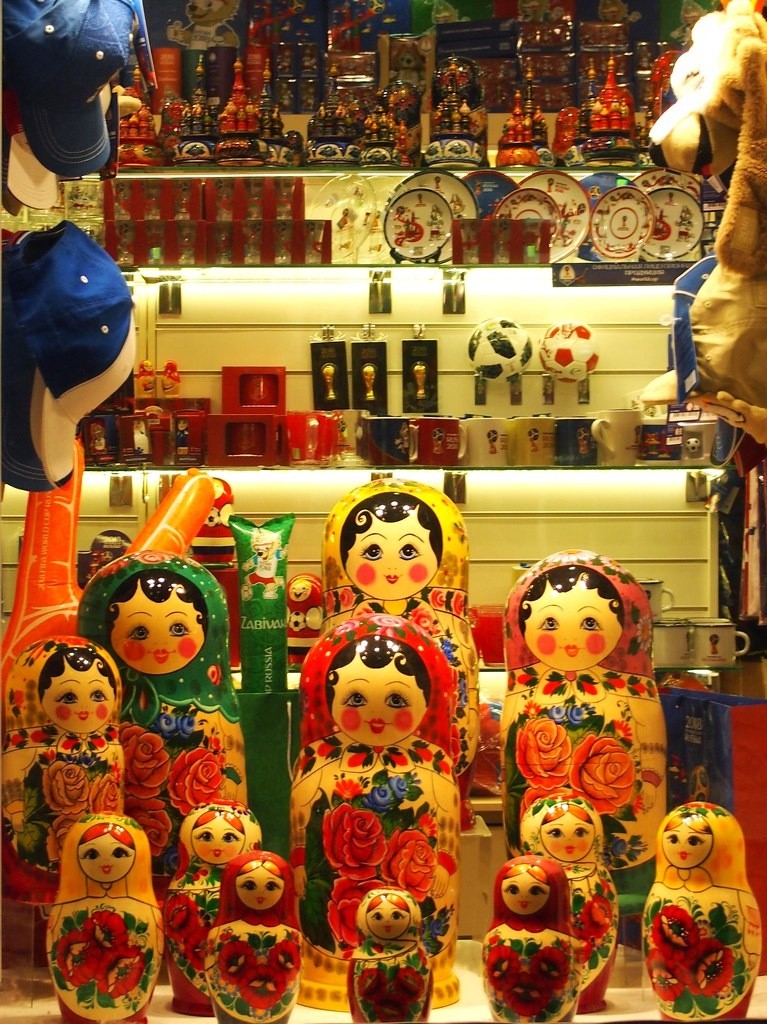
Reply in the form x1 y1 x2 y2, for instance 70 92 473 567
205 414 276 467
221 365 287 416
324 80 378 111
116 413 152 465
324 50 380 82
434 17 638 114
458 814 493 940
103 178 333 266
78 414 119 466
135 397 211 414
274 415 292 468
150 411 205 467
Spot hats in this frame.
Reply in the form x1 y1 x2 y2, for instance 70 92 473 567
2 221 137 491
2 0 134 177
1 84 59 210
1 129 24 216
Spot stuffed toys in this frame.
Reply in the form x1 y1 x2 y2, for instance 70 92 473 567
648 0 767 274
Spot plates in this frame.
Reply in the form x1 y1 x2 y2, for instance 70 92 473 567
302 169 705 260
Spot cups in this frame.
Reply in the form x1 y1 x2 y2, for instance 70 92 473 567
651 621 691 667
694 623 750 667
637 577 675 621
361 408 716 467
470 605 506 668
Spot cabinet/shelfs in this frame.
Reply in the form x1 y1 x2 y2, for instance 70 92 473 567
0 164 767 676
